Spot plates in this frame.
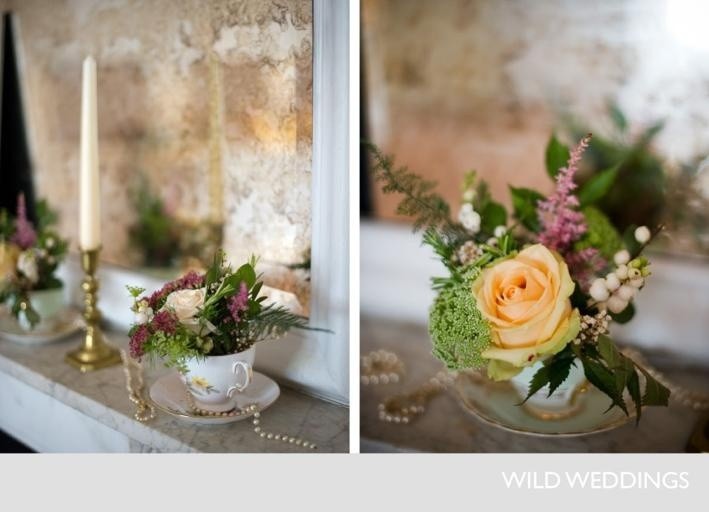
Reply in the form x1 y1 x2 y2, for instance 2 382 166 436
453 372 651 441
0 307 82 345
149 367 280 427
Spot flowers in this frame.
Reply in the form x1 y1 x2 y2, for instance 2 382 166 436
359 122 674 430
0 192 69 329
122 250 338 369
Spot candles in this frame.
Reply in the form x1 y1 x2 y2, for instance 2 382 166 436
77 55 104 251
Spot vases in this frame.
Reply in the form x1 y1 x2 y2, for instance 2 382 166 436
510 354 589 421
7 278 66 333
